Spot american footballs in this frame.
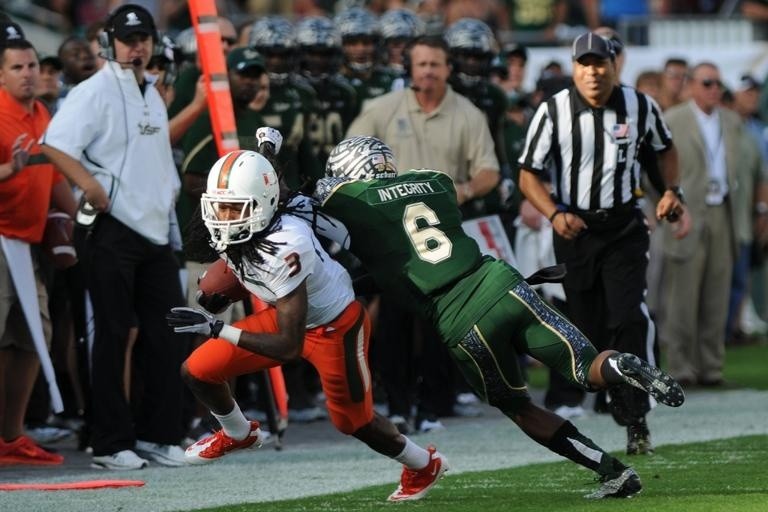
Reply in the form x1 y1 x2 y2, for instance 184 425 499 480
38 208 78 266
196 258 251 303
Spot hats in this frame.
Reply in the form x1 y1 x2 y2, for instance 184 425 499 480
571 32 613 63
595 26 625 48
226 47 268 75
106 4 158 37
1 21 24 46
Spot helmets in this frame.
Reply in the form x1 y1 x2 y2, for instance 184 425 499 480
334 8 383 39
443 17 501 88
298 14 342 51
206 149 281 232
248 15 301 52
379 8 433 41
325 135 399 181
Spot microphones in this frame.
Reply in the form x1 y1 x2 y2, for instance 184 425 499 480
402 73 421 93
97 38 143 67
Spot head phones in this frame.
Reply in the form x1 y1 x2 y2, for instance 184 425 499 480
97 5 162 49
401 34 462 78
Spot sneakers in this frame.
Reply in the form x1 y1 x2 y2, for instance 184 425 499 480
383 414 410 435
25 417 73 446
181 422 263 465
616 352 687 408
1 435 64 467
542 395 585 421
146 441 210 468
581 467 642 499
414 415 448 436
387 446 450 503
90 450 148 471
285 400 327 422
625 417 652 457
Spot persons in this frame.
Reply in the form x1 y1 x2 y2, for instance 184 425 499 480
2 1 767 504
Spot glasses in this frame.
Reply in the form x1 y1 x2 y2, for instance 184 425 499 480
223 36 237 46
694 78 722 88
